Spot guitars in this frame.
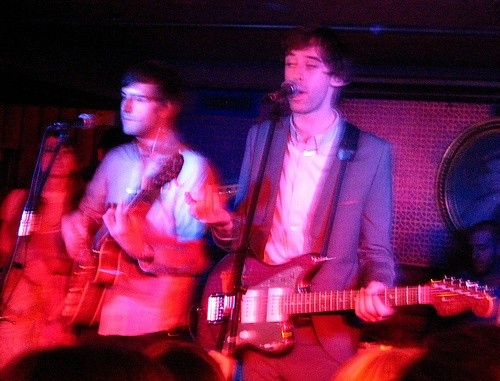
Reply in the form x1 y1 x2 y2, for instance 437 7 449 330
196 251 498 360
61 154 184 327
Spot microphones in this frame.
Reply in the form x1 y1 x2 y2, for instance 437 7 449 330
49 112 97 133
267 81 300 101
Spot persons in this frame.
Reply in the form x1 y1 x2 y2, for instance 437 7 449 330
0 130 87 371
60 71 220 350
184 28 397 380
445 219 499 328
